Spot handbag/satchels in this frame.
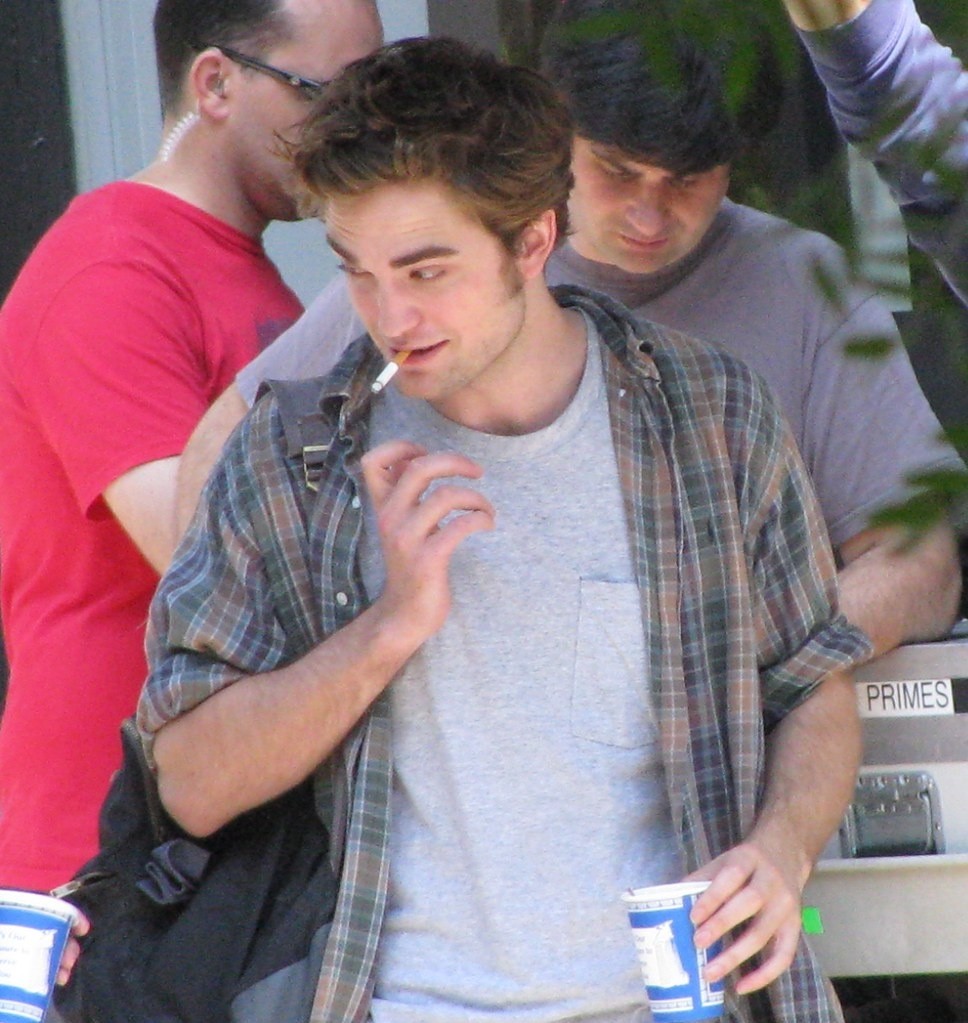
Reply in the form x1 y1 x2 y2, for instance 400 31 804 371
40 714 344 1023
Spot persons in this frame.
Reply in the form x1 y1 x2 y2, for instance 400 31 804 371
0 0 385 896
55 909 89 986
137 0 968 1023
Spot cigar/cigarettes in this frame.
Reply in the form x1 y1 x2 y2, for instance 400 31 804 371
371 352 412 396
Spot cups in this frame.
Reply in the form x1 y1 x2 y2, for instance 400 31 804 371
0 890 80 1023
621 881 724 1023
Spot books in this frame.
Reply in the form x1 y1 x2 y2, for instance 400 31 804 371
0 890 78 1023
